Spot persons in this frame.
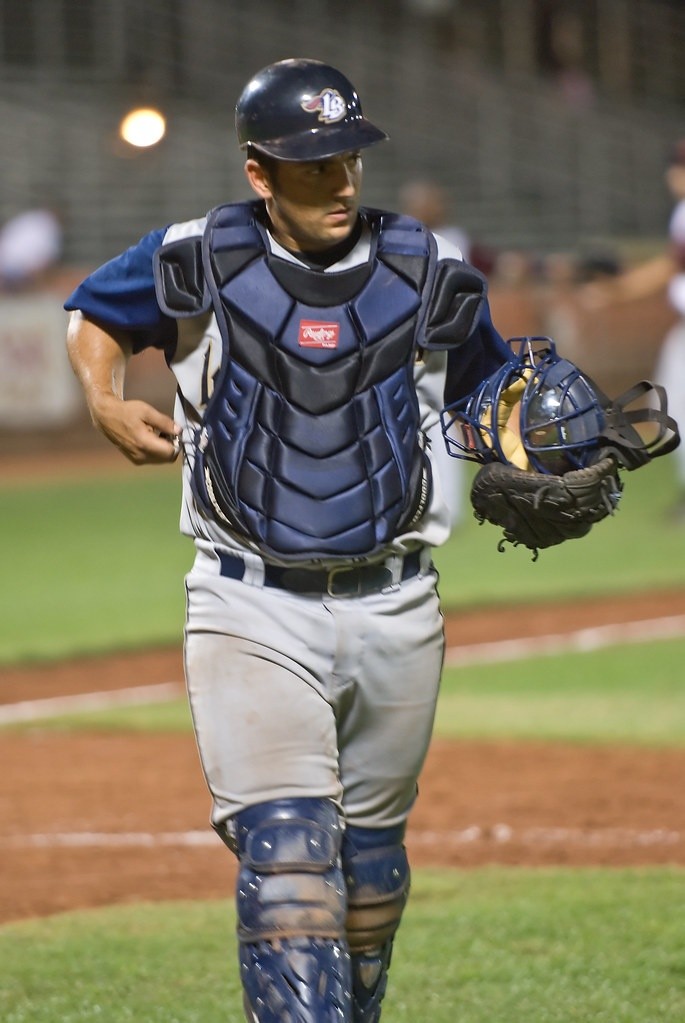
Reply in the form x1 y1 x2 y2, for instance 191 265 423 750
587 149 685 485
398 177 498 545
63 57 622 1022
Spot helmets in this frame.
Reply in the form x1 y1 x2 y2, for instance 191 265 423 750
234 57 392 163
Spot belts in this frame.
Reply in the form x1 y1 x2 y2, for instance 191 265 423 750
212 549 421 600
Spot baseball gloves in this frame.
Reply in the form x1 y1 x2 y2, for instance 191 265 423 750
460 357 627 567
